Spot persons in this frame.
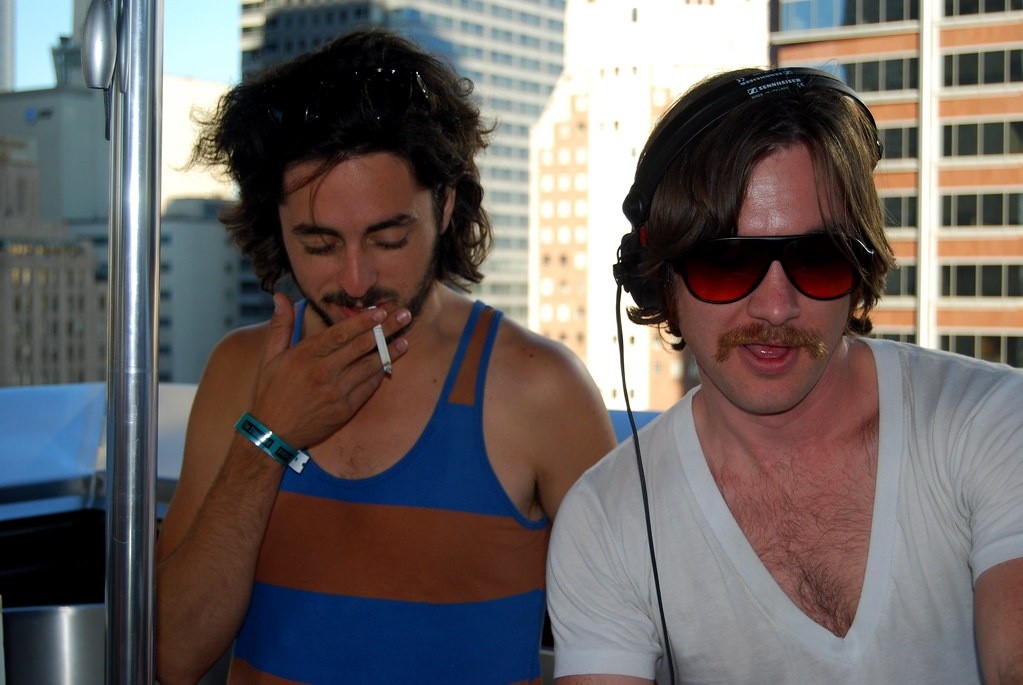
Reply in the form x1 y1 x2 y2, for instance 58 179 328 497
547 68 1023 685
155 30 619 685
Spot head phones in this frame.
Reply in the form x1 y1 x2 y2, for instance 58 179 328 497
613 67 883 314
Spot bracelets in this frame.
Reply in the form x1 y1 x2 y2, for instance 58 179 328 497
233 412 310 473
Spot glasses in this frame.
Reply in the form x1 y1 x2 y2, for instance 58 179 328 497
263 68 452 134
669 233 875 304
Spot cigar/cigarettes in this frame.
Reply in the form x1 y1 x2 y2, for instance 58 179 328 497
369 305 392 374
